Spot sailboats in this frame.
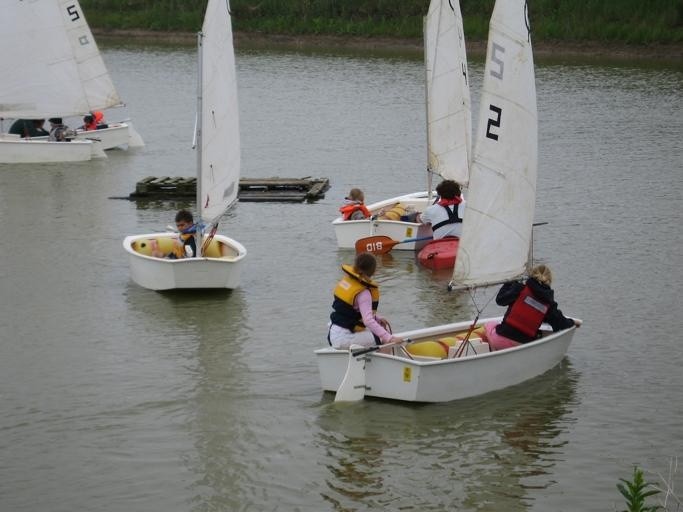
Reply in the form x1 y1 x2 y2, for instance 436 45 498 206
315 0 581 403
0 0 144 163
332 1 473 251
123 1 247 292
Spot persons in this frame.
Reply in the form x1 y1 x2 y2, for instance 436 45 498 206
327 252 403 354
81 116 97 131
417 180 466 242
163 210 203 259
481 264 582 352
339 188 385 220
45 118 65 142
7 118 49 138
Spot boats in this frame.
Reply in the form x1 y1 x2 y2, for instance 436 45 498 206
130 176 330 201
417 240 462 269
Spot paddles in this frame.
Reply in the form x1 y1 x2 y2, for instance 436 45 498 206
355 222 548 255
